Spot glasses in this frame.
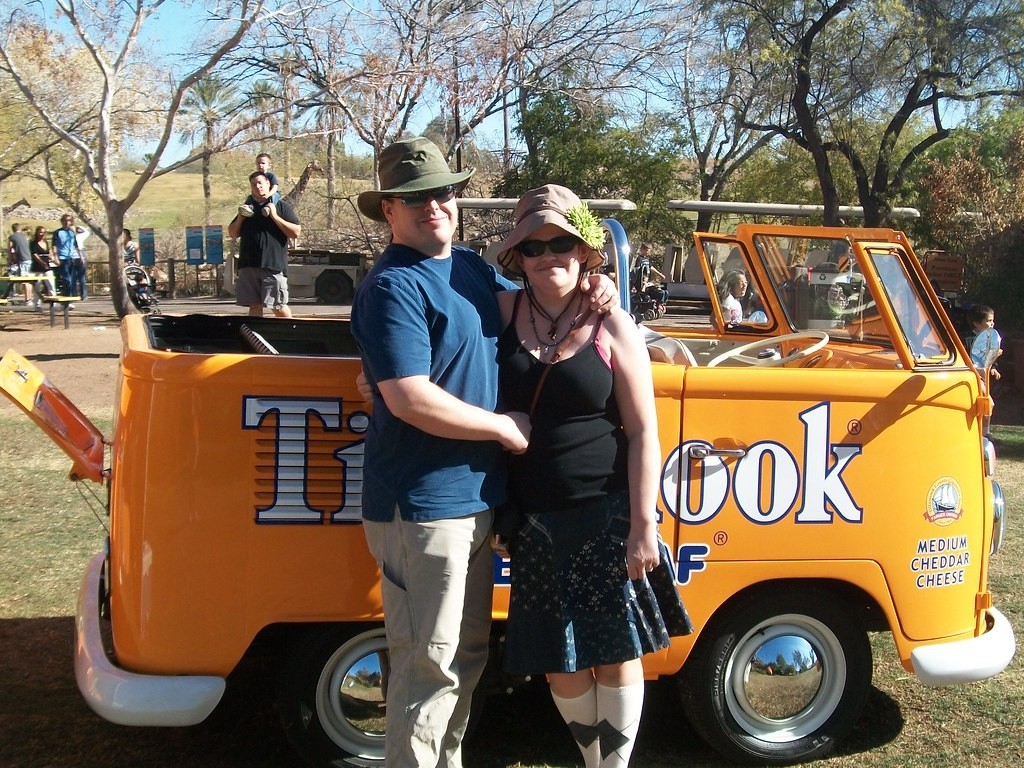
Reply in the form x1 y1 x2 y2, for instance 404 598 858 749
386 184 454 208
515 234 583 257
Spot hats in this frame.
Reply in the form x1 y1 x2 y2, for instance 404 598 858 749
357 136 476 222
497 184 605 277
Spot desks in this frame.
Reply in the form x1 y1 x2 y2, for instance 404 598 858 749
0 276 56 311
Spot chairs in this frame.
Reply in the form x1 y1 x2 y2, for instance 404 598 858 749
636 323 699 367
239 323 281 356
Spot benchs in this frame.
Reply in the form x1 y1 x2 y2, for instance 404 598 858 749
43 296 82 329
0 293 33 304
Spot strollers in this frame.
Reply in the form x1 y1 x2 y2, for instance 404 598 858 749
631 265 664 321
124 266 162 314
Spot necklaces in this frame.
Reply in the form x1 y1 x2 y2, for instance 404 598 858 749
527 285 585 355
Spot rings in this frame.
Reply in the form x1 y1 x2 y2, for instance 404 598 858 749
646 567 653 572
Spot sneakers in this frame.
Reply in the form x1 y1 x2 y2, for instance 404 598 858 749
1 289 89 311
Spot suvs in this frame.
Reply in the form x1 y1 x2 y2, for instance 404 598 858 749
224 248 367 305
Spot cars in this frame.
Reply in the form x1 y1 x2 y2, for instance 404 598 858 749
1 200 1018 768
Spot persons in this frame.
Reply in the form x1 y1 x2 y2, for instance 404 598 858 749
639 271 656 294
30 226 59 311
8 222 34 307
117 229 141 280
964 304 1003 443
238 153 281 217
349 137 532 768
709 269 748 331
133 274 149 300
68 226 89 301
52 214 85 311
228 171 301 319
477 185 694 768
635 242 665 283
